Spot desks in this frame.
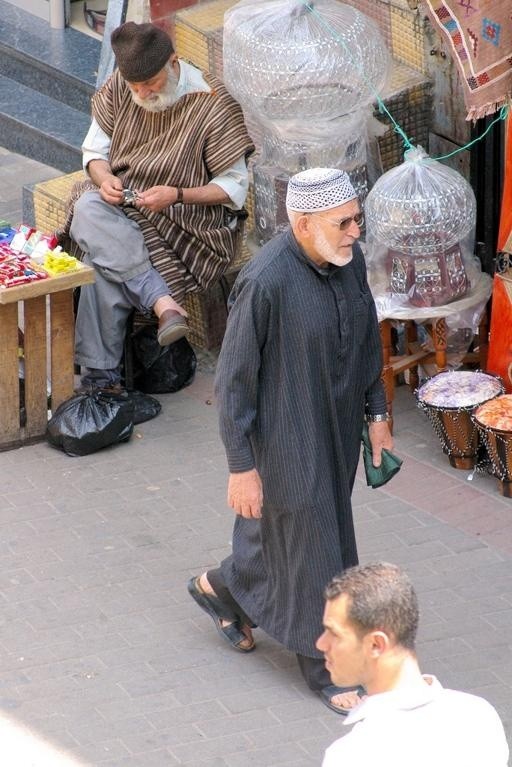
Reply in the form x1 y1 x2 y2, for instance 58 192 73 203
1 236 95 453
372 260 491 437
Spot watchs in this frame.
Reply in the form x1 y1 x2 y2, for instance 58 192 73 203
172 184 185 209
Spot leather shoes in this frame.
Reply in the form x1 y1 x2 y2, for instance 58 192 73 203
45 382 122 410
157 308 190 347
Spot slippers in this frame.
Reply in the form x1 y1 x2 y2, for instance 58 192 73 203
187 573 255 654
316 677 366 720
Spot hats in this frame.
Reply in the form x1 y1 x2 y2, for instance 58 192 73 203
110 21 173 82
285 166 358 214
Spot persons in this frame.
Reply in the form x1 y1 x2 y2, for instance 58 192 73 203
70 20 256 390
187 166 394 717
314 560 511 767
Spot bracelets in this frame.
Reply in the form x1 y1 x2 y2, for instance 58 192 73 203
365 413 391 424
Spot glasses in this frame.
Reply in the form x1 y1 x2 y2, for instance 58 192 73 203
301 212 363 231
